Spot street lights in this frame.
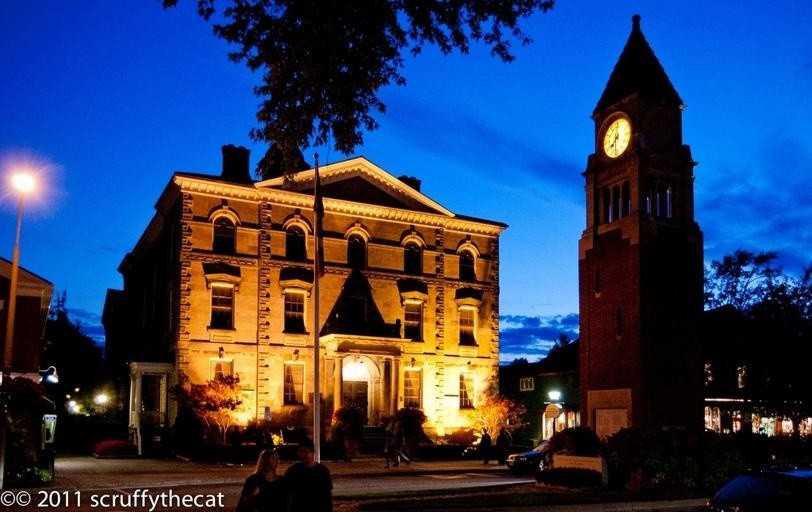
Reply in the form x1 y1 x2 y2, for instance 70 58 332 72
3 169 39 372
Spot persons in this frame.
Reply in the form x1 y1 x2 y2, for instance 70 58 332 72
237 437 333 512
496 426 511 465
479 429 491 464
332 421 352 464
383 421 410 468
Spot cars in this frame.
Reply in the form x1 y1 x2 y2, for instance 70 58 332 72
505 440 552 474
706 462 812 512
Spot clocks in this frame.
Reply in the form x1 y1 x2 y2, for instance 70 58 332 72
601 118 632 160
